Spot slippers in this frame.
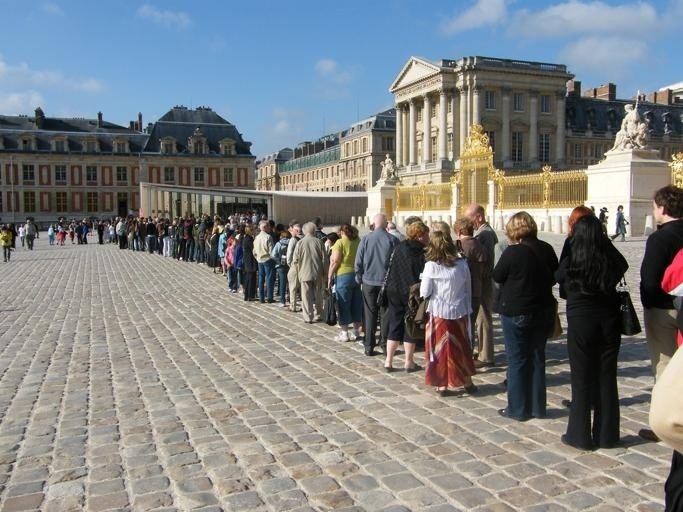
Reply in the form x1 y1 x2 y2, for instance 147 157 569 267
465 383 477 393
405 364 422 372
435 387 446 398
385 365 392 373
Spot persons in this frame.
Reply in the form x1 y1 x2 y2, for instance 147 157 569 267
107 207 266 268
598 206 608 236
661 249 683 351
224 204 497 399
491 211 560 418
661 294 683 511
379 153 399 180
638 184 682 443
609 206 629 243
556 217 631 451
555 207 604 410
620 91 646 150
0 213 105 263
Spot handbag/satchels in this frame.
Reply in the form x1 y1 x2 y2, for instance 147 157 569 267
323 286 337 326
618 290 642 335
376 283 388 307
548 297 561 339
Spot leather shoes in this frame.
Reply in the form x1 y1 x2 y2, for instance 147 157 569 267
365 351 373 356
473 359 495 368
497 409 525 421
639 428 662 442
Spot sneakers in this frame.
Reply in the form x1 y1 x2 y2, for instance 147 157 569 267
350 331 360 341
334 331 349 342
